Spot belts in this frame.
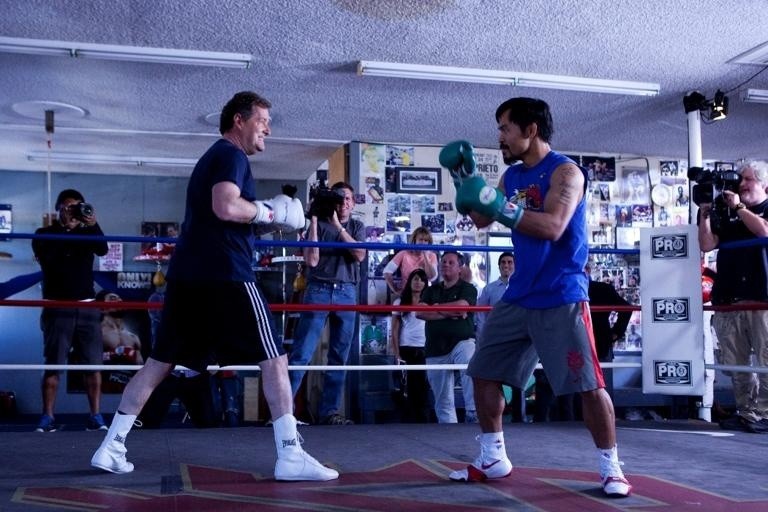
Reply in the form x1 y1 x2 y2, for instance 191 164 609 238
307 275 356 291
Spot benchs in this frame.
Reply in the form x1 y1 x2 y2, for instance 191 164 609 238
358 386 465 426
611 383 688 421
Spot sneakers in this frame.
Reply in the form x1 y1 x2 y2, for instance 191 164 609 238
82 412 110 435
719 413 764 436
33 412 62 435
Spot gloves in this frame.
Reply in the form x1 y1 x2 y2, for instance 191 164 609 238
453 175 527 232
249 186 307 234
435 138 479 182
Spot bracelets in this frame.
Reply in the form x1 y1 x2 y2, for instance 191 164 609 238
336 226 347 232
733 202 746 211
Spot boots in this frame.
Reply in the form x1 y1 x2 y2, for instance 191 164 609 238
267 412 341 483
592 441 634 499
88 410 140 476
445 432 520 484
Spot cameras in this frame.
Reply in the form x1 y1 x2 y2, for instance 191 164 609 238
68 202 94 221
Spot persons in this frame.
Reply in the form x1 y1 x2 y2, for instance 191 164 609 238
697 159 768 434
162 226 179 256
415 252 477 424
586 264 633 403
96 289 146 392
134 281 219 428
367 228 382 270
474 253 520 335
391 268 430 425
438 99 632 497
146 223 155 252
33 191 110 434
89 92 340 481
288 181 367 424
584 157 689 304
384 227 438 294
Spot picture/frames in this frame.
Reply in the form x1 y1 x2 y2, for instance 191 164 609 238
392 165 443 195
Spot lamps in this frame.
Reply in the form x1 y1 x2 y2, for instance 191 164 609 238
71 40 253 71
355 58 518 90
681 88 730 125
737 86 767 104
0 36 75 62
515 69 662 100
